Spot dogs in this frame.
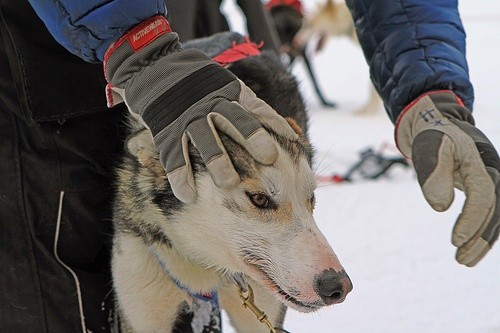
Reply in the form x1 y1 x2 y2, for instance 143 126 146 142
292 2 385 118
115 32 354 333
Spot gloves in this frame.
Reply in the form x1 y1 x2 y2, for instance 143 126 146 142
394 90 500 268
103 16 298 204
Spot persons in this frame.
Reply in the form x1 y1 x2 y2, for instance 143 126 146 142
0 0 500 332
